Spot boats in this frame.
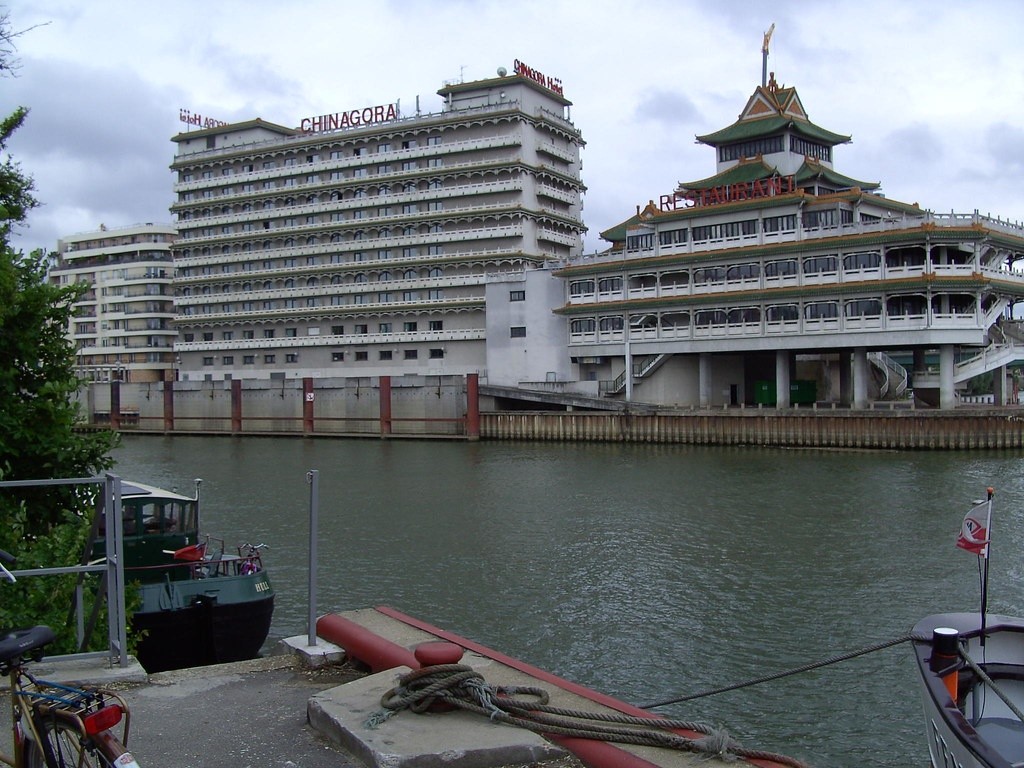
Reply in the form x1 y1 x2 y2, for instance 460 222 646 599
911 484 1024 768
71 475 275 674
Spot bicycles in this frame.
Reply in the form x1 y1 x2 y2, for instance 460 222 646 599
237 542 271 577
0 546 143 768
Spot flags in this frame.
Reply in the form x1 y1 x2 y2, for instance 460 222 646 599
174 542 206 560
956 501 993 556
